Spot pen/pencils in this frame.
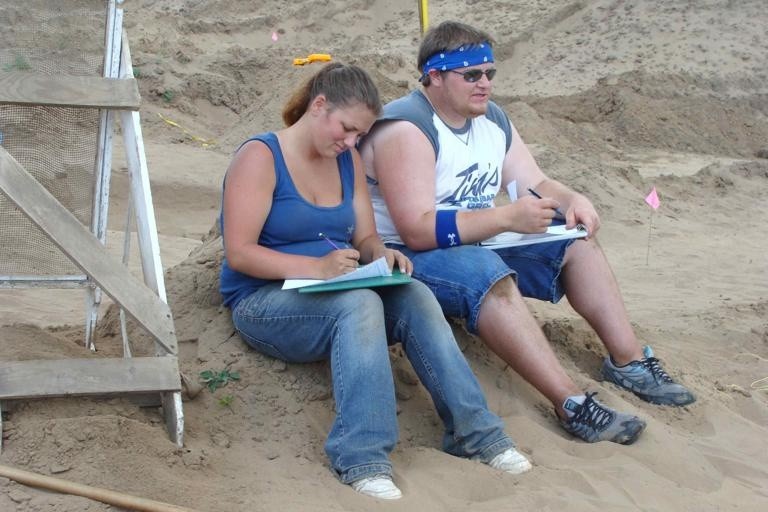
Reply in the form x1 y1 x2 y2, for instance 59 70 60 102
528 189 562 215
318 233 339 250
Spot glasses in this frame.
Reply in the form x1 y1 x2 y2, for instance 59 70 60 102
450 67 496 82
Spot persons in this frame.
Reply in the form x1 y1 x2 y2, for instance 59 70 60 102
360 22 694 445
218 61 533 500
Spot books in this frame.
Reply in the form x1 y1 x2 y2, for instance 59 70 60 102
480 224 588 249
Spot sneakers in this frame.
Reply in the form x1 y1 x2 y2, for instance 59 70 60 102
351 475 403 499
555 390 647 444
490 448 532 474
600 344 696 407
